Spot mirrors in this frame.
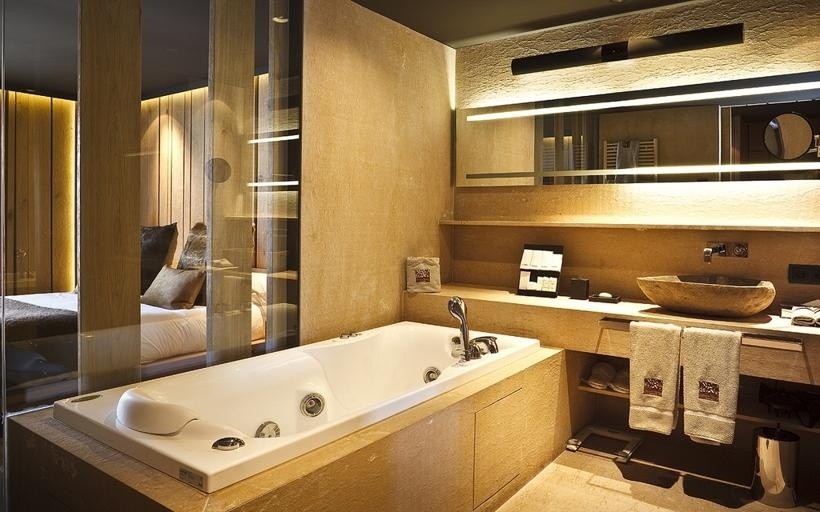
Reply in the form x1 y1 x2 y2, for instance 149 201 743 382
761 110 820 161
454 70 820 189
0 3 302 416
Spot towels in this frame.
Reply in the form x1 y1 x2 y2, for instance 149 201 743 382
628 320 743 448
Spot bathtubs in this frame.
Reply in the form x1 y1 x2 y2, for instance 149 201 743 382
53 319 541 494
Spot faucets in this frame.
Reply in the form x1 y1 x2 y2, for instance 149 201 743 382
447 295 471 361
702 240 728 265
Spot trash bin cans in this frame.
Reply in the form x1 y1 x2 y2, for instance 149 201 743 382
751 427 800 508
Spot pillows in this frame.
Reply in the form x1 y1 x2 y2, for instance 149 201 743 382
140 221 208 309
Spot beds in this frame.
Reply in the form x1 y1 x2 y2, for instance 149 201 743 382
4 289 267 409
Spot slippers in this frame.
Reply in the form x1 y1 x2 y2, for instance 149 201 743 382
588 363 615 389
609 367 630 394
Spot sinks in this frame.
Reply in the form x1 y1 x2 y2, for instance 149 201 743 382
637 270 777 316
